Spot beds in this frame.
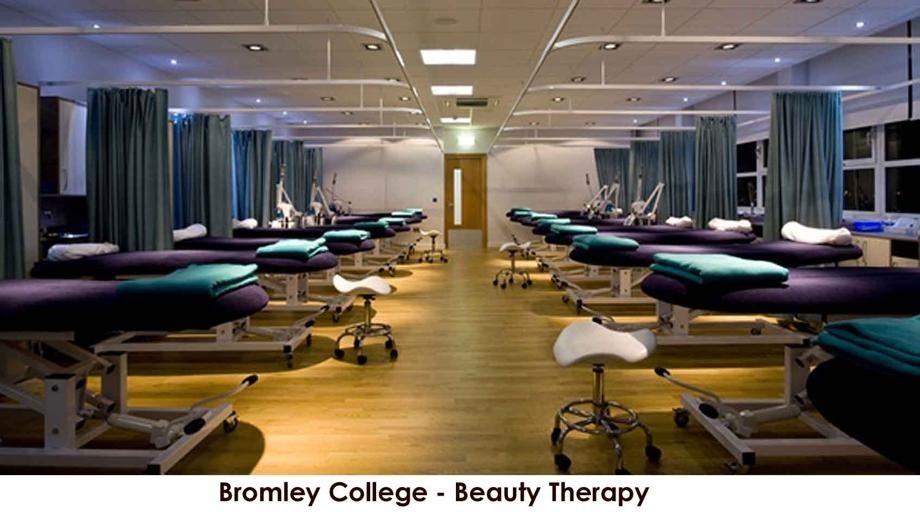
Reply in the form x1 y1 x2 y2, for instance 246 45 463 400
501 209 920 472
0 207 424 475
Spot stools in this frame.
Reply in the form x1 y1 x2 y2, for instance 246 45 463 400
418 227 450 265
493 241 533 289
